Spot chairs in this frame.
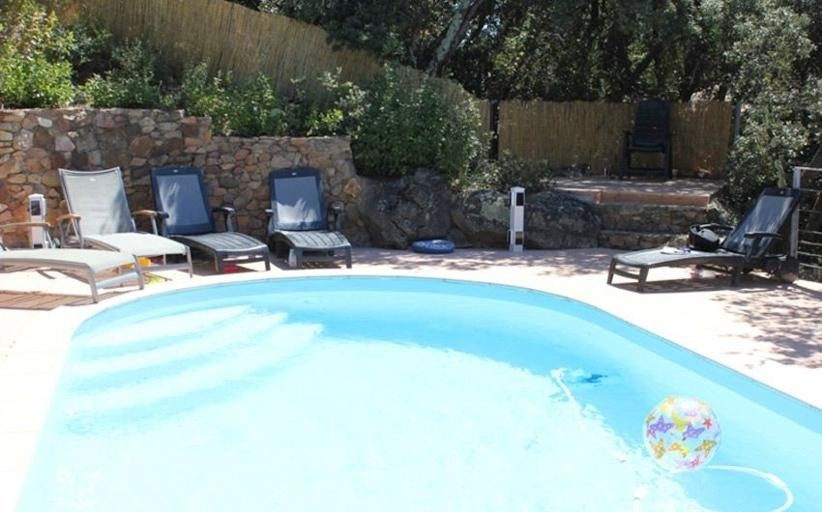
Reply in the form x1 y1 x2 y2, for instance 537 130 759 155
606 185 800 291
622 100 676 180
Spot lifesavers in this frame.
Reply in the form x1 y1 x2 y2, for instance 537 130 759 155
415 241 454 252
